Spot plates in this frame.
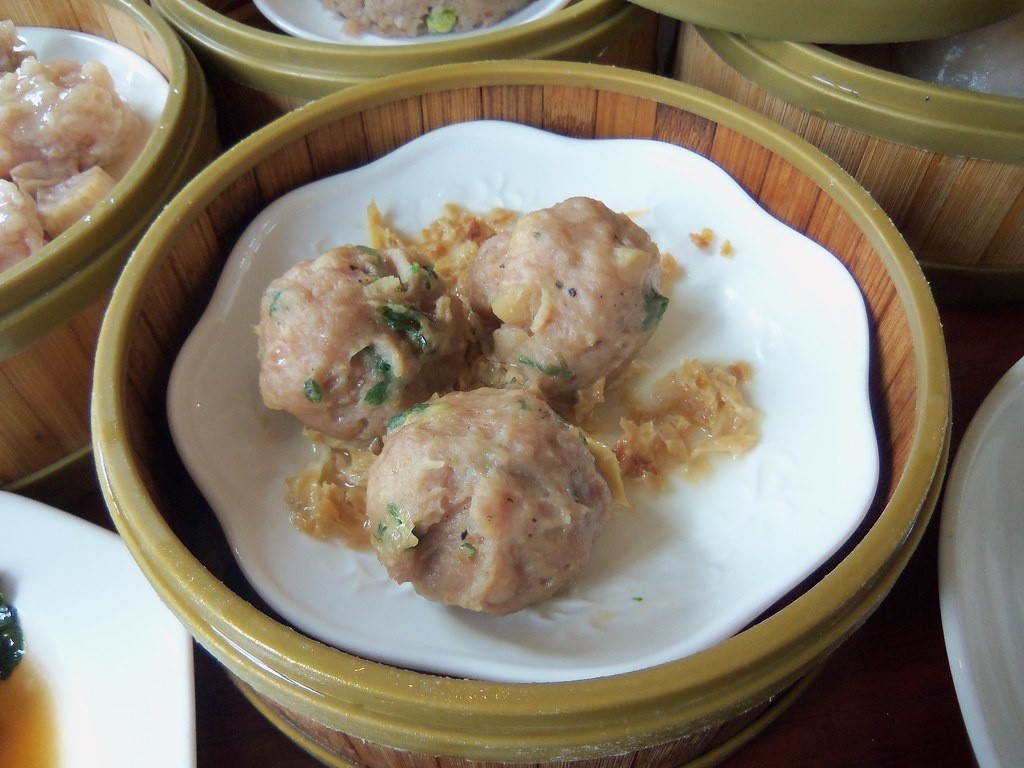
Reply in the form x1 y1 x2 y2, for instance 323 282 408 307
0 490 195 768
0 26 169 183
940 353 1024 768
169 119 882 684
253 0 584 47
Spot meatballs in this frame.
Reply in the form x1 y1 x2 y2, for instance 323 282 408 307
252 194 670 618
